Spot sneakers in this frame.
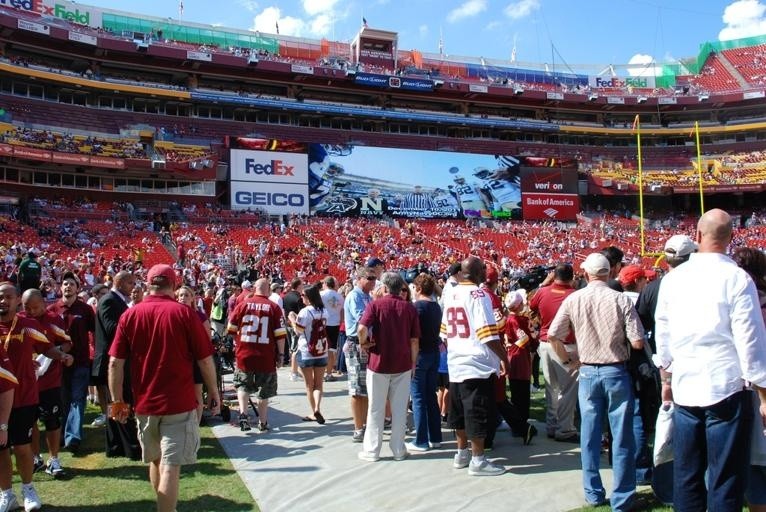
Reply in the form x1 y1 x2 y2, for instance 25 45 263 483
358 451 380 462
467 441 492 452
430 443 441 448
405 442 429 452
20 480 42 512
392 451 410 462
31 453 45 473
523 423 537 445
384 417 392 434
353 428 365 442
453 453 471 469
0 487 19 512
238 413 251 431
257 420 269 431
44 456 67 480
468 460 506 477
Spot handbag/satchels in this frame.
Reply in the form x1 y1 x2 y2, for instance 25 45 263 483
652 402 674 467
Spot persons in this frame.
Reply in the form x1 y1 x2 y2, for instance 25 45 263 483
308 143 521 220
576 139 766 187
0 199 764 510
0 101 219 164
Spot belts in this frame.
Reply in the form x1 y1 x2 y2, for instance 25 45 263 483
347 335 369 343
582 361 623 366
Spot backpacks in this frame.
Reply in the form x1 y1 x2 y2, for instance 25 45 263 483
304 310 333 357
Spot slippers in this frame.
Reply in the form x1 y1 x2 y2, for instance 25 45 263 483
314 412 325 425
302 416 316 421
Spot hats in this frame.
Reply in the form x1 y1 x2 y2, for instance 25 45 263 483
663 234 699 258
485 265 498 285
324 275 334 282
618 263 656 285
61 269 80 289
146 261 177 286
270 282 283 290
502 287 527 309
367 257 384 267
579 252 611 277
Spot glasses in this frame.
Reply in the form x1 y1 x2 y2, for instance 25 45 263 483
402 287 407 292
361 275 377 281
